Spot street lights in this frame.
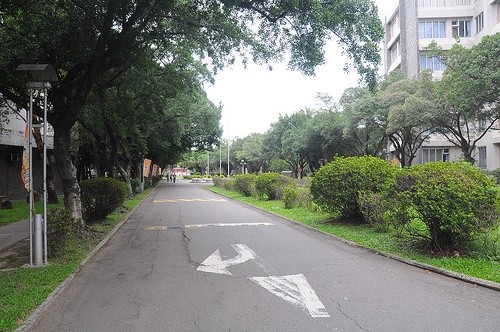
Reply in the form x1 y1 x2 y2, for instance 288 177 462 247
16 63 60 266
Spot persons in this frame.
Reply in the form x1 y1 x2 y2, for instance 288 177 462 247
167 173 170 182
170 173 173 182
173 173 176 183
